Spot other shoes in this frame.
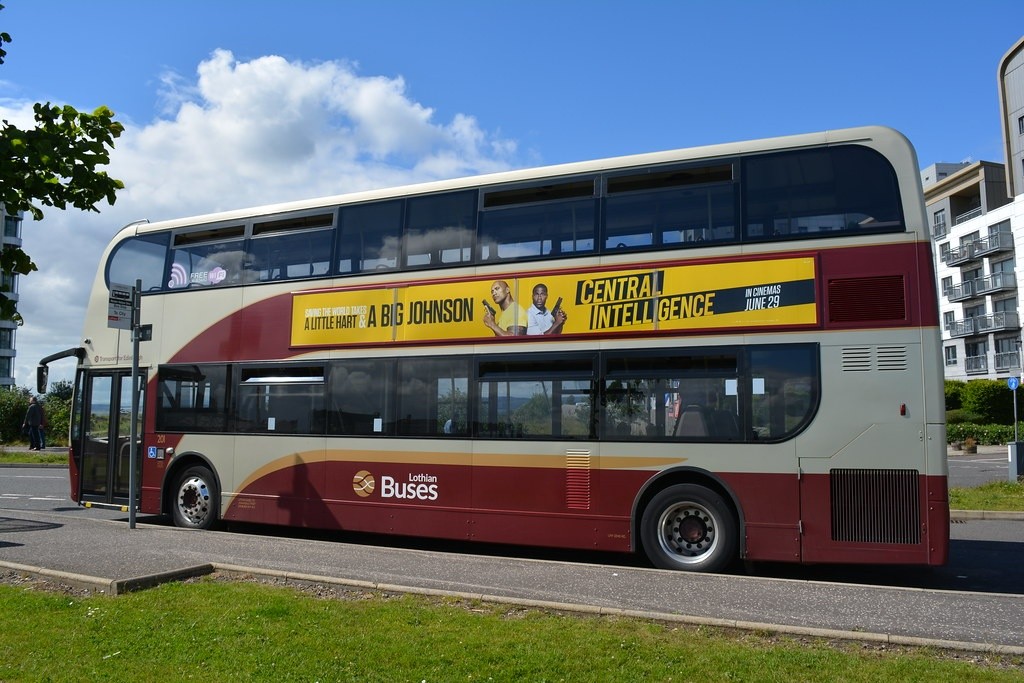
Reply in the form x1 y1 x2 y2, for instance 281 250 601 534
32 448 40 451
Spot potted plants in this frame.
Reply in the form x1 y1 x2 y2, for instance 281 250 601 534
966 438 977 453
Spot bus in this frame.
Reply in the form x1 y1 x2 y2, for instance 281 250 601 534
37 126 949 572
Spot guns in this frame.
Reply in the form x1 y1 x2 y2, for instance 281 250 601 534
551 297 564 317
482 298 497 316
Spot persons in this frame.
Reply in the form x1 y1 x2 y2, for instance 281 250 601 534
38 409 49 449
527 283 567 335
22 397 43 451
481 280 527 337
